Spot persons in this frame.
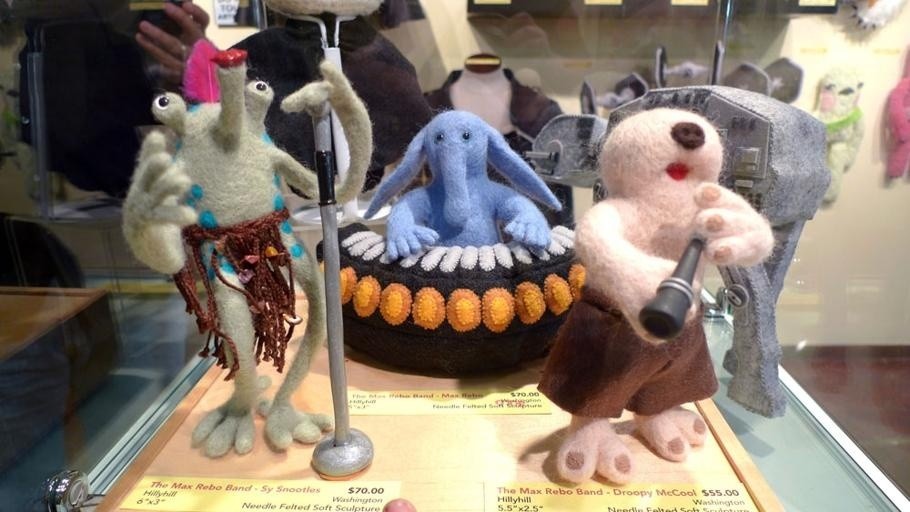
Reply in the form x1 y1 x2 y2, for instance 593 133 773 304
419 52 577 232
16 1 209 200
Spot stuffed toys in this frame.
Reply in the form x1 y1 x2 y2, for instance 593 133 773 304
882 47 910 177
223 0 433 201
524 81 833 416
813 68 867 203
537 109 773 487
123 48 371 459
363 110 563 264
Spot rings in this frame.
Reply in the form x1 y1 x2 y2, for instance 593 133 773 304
181 44 187 61
